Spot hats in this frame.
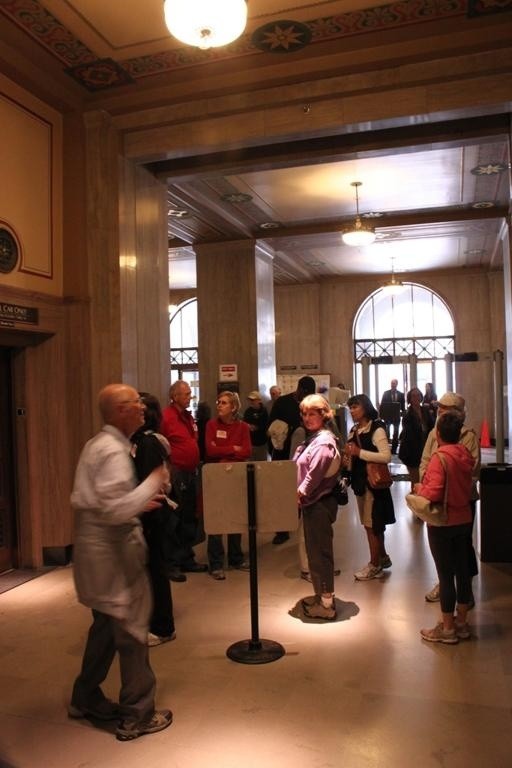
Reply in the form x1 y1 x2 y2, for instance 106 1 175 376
434 392 465 411
268 420 289 450
247 391 261 400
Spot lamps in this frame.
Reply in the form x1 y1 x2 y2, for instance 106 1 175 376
380 255 405 296
340 183 377 246
163 1 248 49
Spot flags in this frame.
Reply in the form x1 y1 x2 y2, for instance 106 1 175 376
480 418 492 448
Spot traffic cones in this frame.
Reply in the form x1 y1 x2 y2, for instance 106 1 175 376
480 416 493 448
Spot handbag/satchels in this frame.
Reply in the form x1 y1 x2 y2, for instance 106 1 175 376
406 494 448 525
367 461 393 489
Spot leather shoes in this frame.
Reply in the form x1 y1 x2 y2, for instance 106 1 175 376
170 536 290 582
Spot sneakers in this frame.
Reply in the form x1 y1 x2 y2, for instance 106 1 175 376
68 630 177 741
300 555 474 644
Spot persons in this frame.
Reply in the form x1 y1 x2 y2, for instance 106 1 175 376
129 393 177 647
345 394 396 580
291 394 341 620
158 380 209 583
66 385 173 742
398 382 478 644
204 377 350 585
379 379 405 455
